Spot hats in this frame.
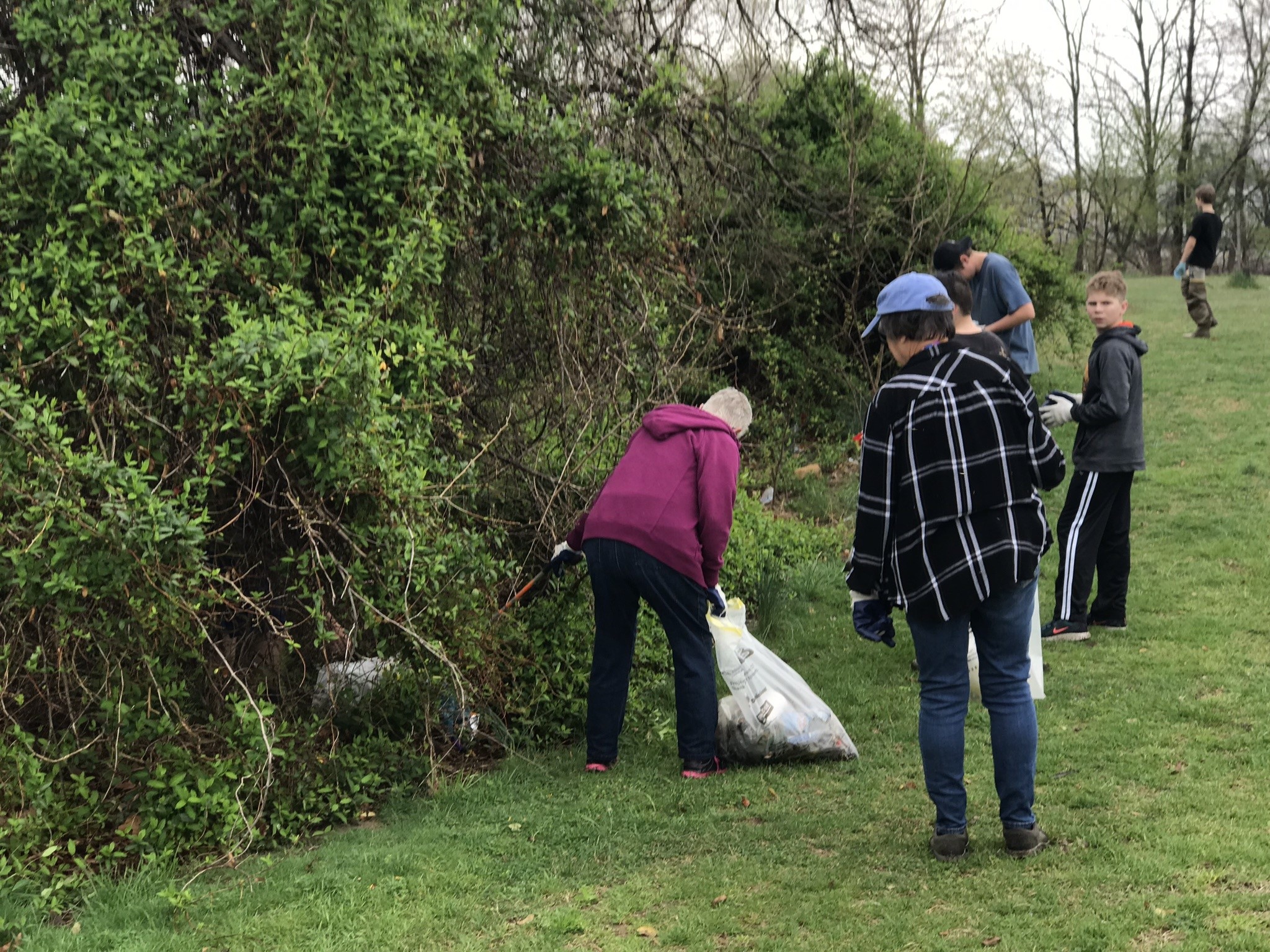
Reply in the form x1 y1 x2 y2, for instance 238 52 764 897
859 270 953 339
932 235 973 271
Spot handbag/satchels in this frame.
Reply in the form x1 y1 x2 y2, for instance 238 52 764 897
706 596 861 764
964 583 1044 702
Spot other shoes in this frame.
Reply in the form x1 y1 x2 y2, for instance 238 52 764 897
1185 316 1217 340
681 752 730 778
584 756 617 772
1001 819 1052 859
926 821 970 860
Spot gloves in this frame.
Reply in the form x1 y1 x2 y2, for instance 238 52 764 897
542 538 583 579
1172 261 1187 280
849 589 896 650
1036 389 1083 431
707 581 729 618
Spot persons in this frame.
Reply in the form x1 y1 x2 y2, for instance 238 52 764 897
846 272 1068 860
1173 184 1222 339
549 387 753 778
1038 269 1149 641
932 236 1040 394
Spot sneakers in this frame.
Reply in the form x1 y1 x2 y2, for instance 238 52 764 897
1086 612 1127 631
1041 620 1091 642
